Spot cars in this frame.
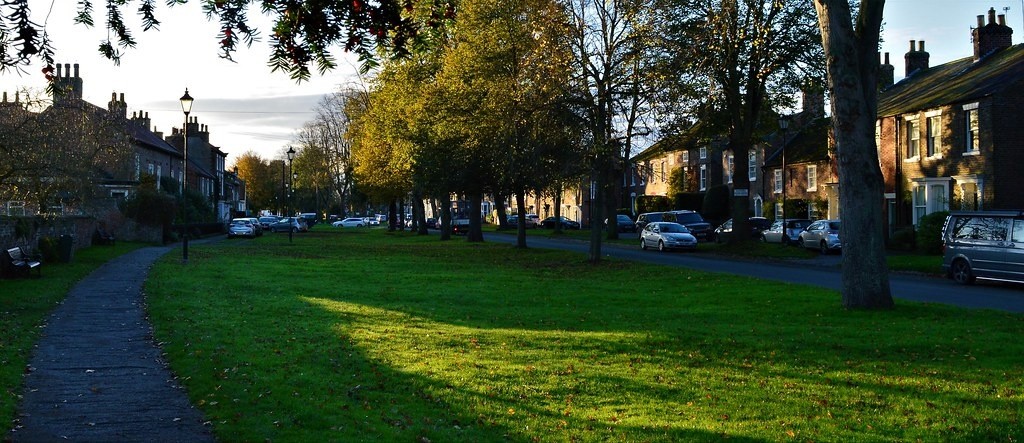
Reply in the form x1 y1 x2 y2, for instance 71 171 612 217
248 218 263 236
259 216 279 230
640 221 698 251
541 216 580 230
364 217 380 226
403 214 419 228
228 218 256 239
508 217 538 229
604 215 636 233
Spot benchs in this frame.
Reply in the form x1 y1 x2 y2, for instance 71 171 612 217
96 228 116 246
3 246 42 280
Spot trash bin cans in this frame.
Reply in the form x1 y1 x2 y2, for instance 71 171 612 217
39 235 73 264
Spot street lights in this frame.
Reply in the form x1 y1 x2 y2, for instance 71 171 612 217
779 112 790 244
285 147 298 243
180 87 193 261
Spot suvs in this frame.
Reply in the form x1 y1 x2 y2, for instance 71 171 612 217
332 218 365 228
662 210 712 243
269 217 308 233
760 219 812 244
712 217 770 244
526 214 541 225
634 212 662 233
798 220 842 255
387 214 400 228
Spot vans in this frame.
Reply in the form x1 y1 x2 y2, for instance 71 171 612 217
941 211 1024 284
376 215 386 223
427 218 437 228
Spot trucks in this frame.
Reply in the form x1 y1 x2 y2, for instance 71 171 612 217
438 207 469 235
